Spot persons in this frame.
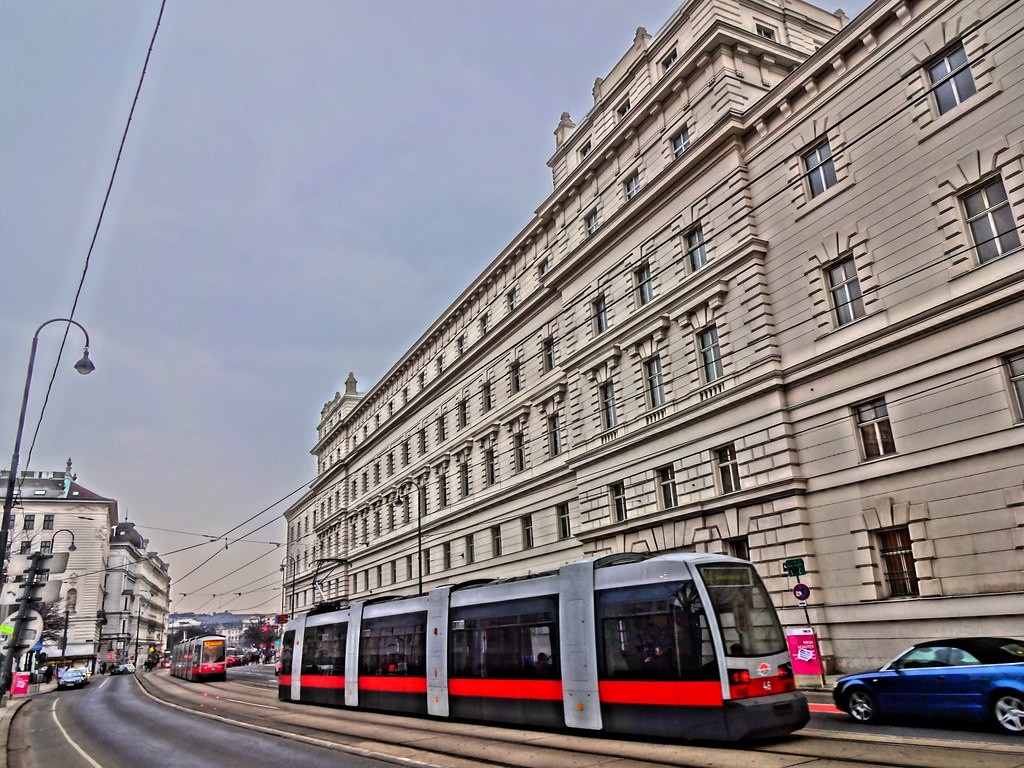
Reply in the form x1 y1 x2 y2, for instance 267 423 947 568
44 664 53 684
110 661 122 670
241 651 270 666
143 657 154 673
534 653 551 677
101 661 108 675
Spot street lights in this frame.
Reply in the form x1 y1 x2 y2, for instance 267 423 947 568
280 555 296 620
134 603 148 668
0 318 96 595
27 528 77 684
394 480 423 596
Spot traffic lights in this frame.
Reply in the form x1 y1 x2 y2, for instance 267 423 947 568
264 627 268 631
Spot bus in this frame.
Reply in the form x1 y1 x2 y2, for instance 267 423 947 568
169 633 227 682
278 551 813 746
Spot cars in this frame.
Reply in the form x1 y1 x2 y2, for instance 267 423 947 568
226 647 269 667
831 636 1024 736
57 667 92 691
111 663 136 675
